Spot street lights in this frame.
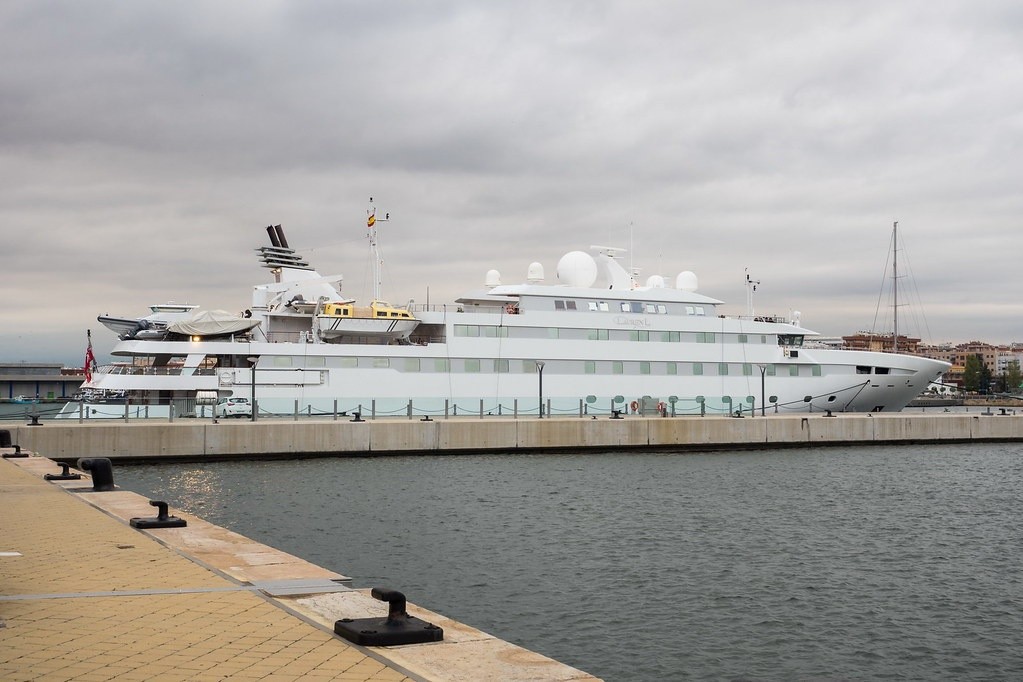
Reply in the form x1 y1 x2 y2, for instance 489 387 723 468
247 357 260 419
536 361 547 417
758 364 766 416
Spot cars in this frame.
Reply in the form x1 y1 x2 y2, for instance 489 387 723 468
216 397 252 418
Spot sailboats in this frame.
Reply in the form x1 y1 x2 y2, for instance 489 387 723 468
858 221 959 397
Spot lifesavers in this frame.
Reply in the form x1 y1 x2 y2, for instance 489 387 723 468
506 304 515 314
630 401 639 411
657 402 667 412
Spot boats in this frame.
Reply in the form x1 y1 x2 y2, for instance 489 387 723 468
318 302 421 341
54 223 952 421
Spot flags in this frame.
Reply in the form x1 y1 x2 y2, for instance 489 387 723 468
84 344 94 383
